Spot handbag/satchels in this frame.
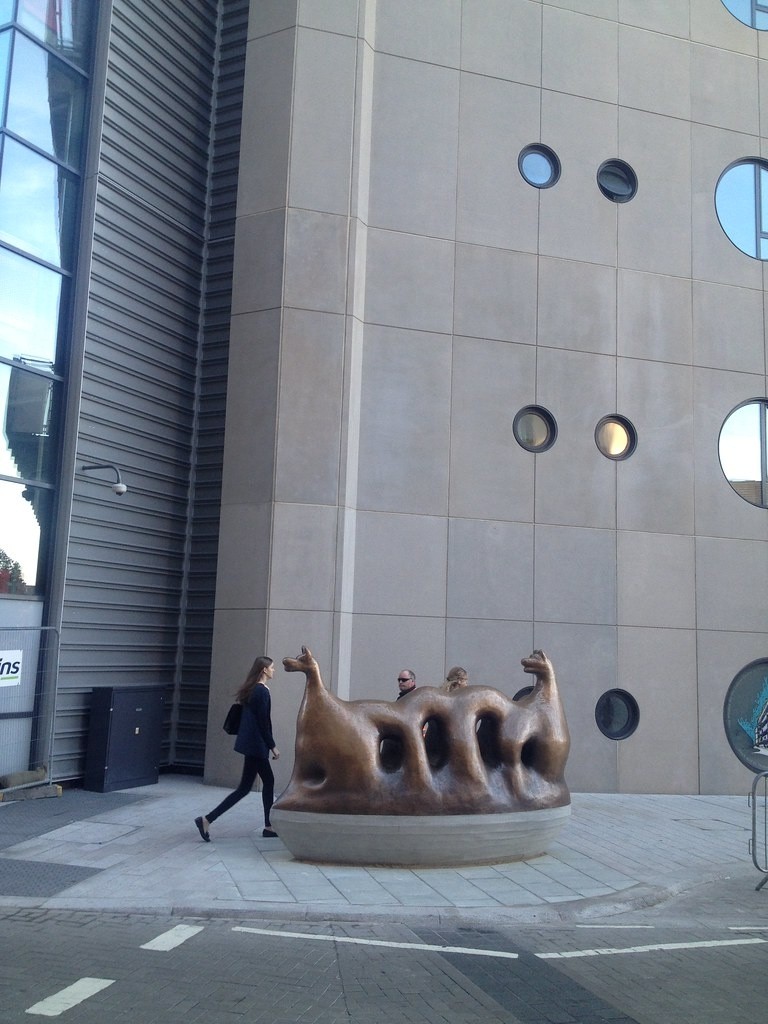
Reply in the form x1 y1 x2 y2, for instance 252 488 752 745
223 703 243 736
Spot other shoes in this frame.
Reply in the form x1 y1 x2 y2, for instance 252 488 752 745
263 829 279 837
194 817 211 841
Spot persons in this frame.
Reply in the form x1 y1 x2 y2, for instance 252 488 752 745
380 668 416 763
192 656 280 842
423 666 469 770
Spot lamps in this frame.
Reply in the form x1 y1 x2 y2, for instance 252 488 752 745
82 465 127 496
22 490 30 501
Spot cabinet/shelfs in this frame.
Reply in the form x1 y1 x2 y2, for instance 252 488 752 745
82 686 166 794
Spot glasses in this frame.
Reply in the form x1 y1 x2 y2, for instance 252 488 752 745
398 678 412 682
